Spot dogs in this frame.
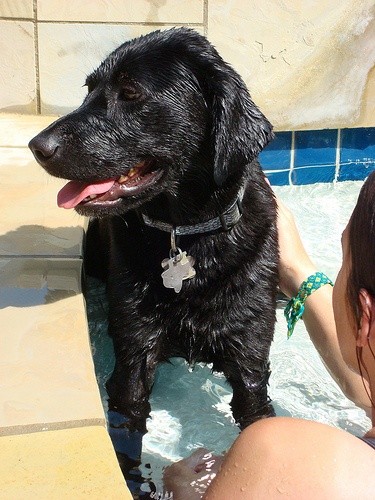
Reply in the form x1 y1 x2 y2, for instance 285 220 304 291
28 26 280 500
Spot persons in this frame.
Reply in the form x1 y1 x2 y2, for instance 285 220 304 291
166 171 375 500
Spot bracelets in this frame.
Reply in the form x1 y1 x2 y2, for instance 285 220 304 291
281 272 338 340
